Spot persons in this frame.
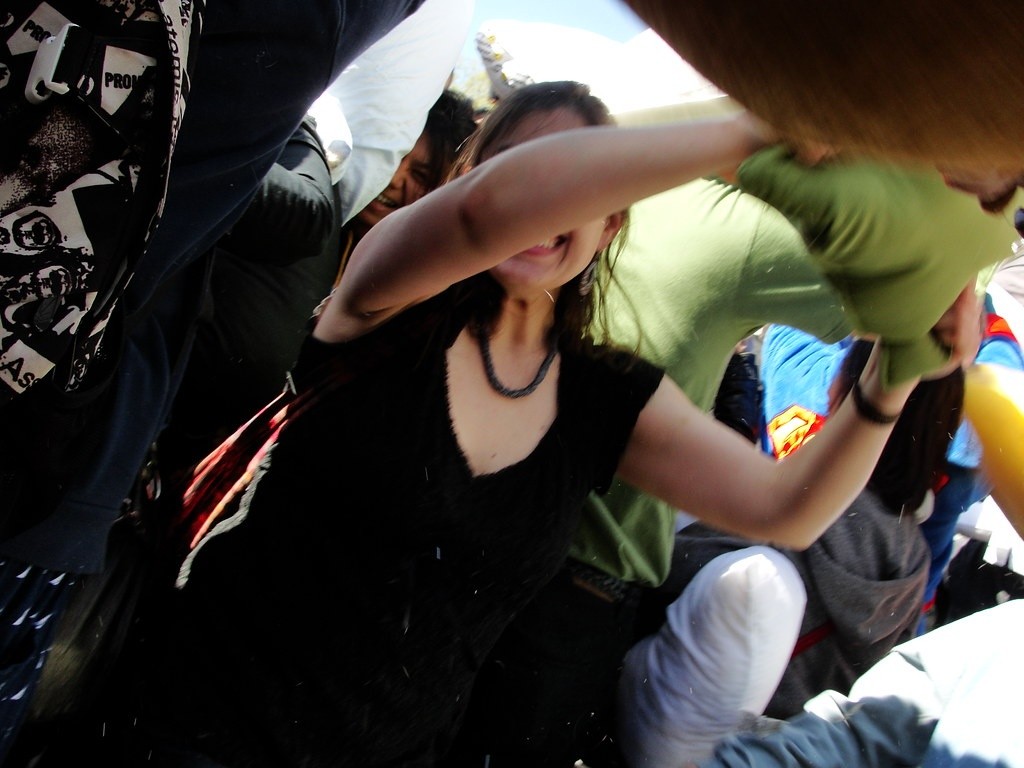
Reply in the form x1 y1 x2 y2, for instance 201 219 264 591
0 0 1024 768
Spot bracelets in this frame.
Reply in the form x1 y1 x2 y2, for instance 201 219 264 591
852 381 903 424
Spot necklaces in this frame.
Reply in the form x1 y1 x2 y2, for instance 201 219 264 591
475 311 559 398
